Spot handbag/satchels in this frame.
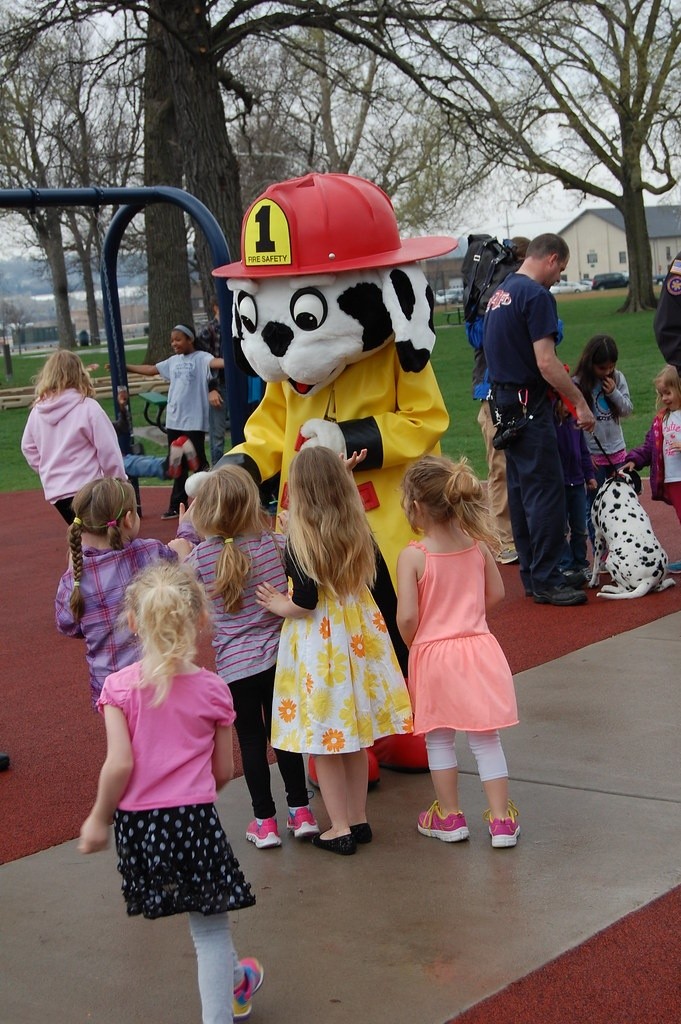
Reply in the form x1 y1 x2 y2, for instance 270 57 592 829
461 234 520 323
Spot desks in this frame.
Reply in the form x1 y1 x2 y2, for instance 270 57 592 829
450 305 465 325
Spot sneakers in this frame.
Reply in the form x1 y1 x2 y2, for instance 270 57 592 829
230 957 265 1018
245 818 283 849
525 574 589 607
417 800 471 843
488 808 522 848
286 807 319 838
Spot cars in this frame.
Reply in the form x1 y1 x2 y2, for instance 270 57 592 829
653 274 666 285
591 272 629 291
549 281 590 293
435 288 464 304
579 278 593 291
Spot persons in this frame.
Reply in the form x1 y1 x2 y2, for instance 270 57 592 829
197 294 230 469
167 459 322 846
53 476 197 699
103 385 199 481
120 436 198 480
255 448 410 855
394 455 520 849
78 563 266 1024
22 350 128 529
106 323 226 519
469 232 681 605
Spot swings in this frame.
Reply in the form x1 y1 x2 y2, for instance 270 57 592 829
25 206 144 482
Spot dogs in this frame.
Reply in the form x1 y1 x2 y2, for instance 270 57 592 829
589 466 677 600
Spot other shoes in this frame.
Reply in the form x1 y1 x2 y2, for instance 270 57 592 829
667 562 681 574
496 546 518 564
160 507 189 520
562 564 608 589
312 821 373 855
258 500 278 516
162 435 199 479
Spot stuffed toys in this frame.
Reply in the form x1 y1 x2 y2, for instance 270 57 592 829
186 171 449 789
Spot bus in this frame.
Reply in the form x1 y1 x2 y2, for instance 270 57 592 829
12 324 76 352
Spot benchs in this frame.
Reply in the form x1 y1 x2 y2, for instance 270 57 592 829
444 310 465 315
138 391 167 431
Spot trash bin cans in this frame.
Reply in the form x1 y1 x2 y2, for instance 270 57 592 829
79 330 89 346
